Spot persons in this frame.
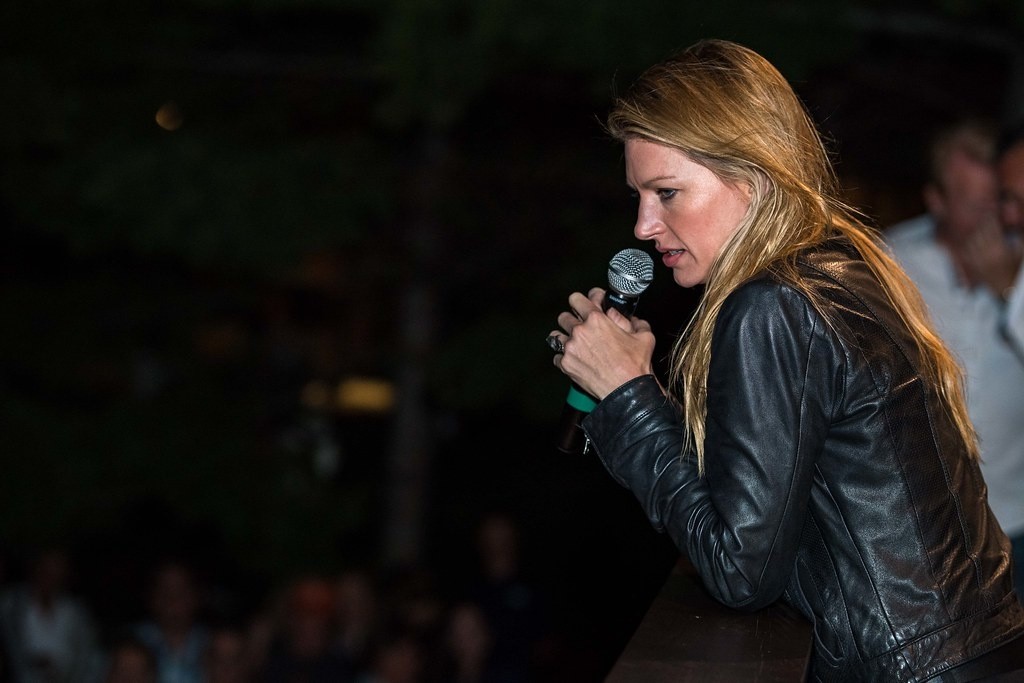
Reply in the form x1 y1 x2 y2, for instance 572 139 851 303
543 40 1023 681
830 108 1022 581
0 508 553 683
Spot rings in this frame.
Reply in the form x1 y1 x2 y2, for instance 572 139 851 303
546 334 564 352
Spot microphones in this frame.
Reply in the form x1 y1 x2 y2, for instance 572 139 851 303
556 248 654 459
